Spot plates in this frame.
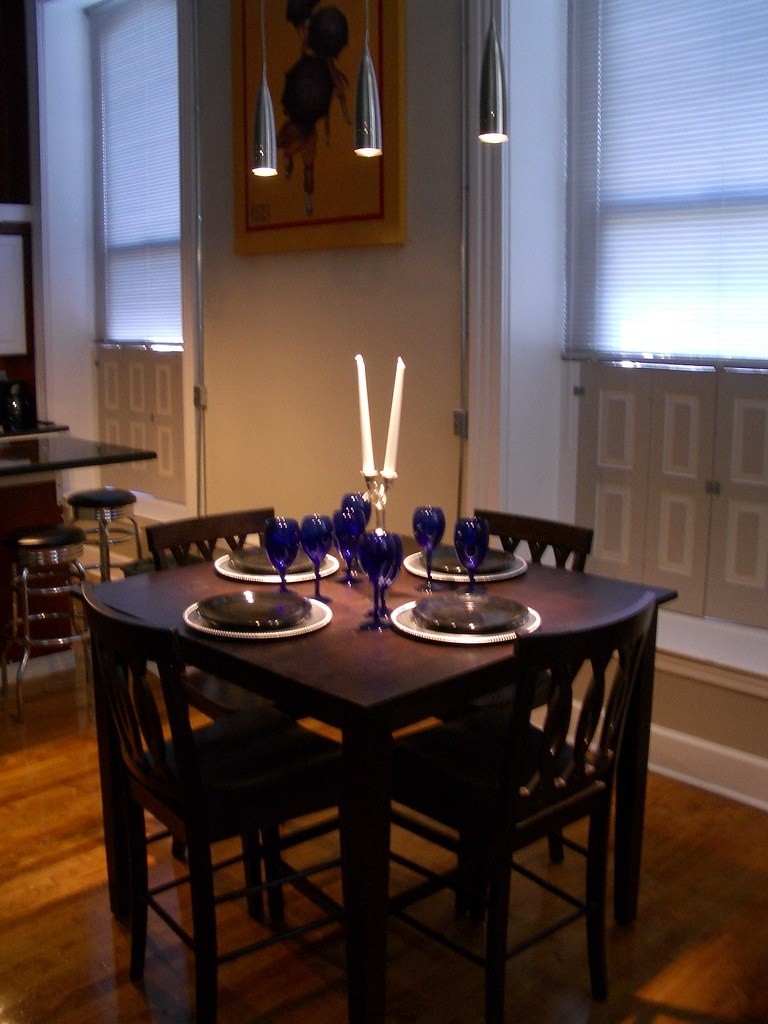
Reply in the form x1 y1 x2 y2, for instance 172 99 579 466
390 601 542 643
196 591 311 630
421 547 513 572
183 599 333 638
414 592 528 632
215 554 340 582
229 546 322 574
404 551 527 582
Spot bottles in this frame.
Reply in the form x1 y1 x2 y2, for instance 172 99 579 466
0 377 33 434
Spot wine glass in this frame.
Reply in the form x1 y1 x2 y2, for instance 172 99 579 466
301 514 334 604
412 507 445 592
264 517 301 595
358 531 404 632
332 492 372 584
455 518 490 595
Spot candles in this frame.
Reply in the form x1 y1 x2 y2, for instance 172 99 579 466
380 356 406 477
355 354 379 478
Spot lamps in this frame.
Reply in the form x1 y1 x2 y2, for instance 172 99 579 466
477 0 510 144
355 0 383 158
252 0 279 177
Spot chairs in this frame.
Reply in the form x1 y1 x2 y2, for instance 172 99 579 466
431 508 595 865
145 505 344 926
80 580 350 1024
389 588 655 1024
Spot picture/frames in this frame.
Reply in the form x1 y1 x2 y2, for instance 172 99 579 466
230 0 405 256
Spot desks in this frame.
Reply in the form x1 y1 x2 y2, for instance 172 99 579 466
69 528 678 1024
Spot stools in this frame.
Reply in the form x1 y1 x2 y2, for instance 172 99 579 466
67 488 144 583
0 523 92 724
